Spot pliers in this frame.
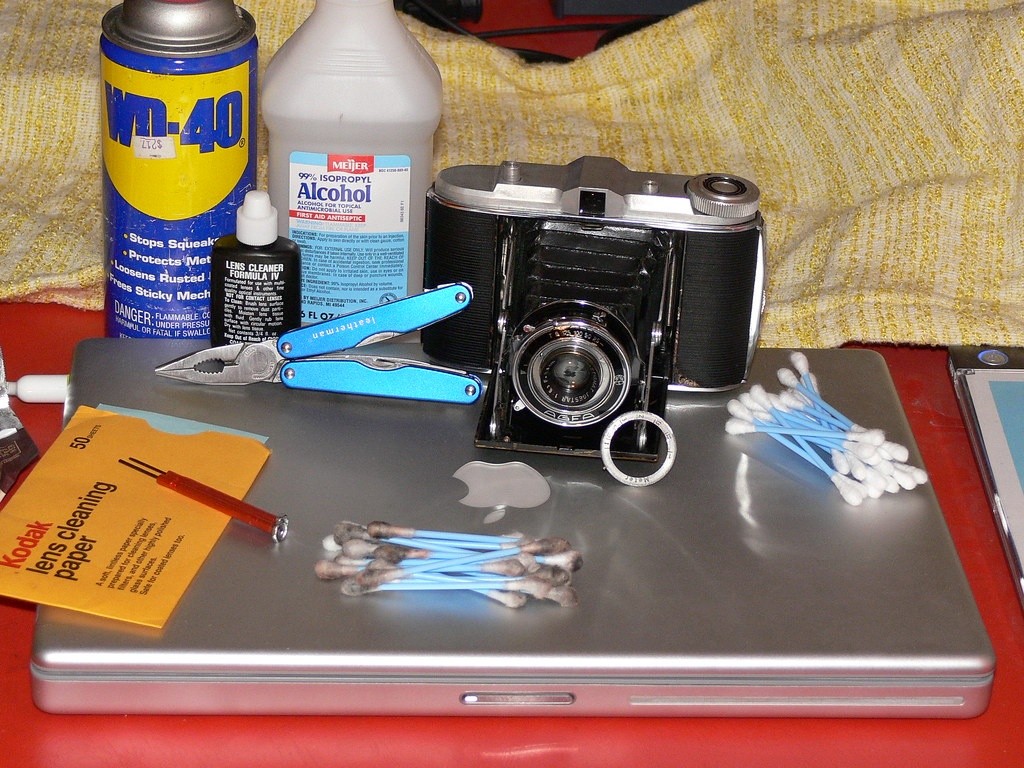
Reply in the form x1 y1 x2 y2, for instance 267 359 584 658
151 279 483 406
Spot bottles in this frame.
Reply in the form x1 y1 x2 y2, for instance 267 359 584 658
100 0 259 339
261 0 443 342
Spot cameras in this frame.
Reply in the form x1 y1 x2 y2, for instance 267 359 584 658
420 156 769 462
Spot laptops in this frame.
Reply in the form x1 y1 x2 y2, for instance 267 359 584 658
946 343 1024 612
29 337 995 718
